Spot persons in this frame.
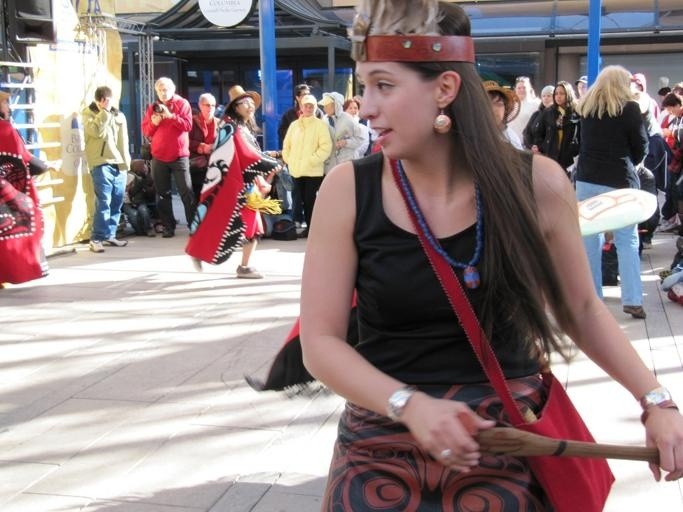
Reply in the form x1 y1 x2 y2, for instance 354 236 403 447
318 91 364 176
575 64 649 319
630 72 683 236
124 159 158 237
506 76 542 139
522 85 555 154
480 80 521 150
658 236 683 307
278 83 329 229
188 91 226 208
188 84 280 279
141 76 197 239
530 81 582 176
82 86 132 252
342 98 373 158
282 94 333 238
297 0 683 511
573 76 588 118
0 90 62 289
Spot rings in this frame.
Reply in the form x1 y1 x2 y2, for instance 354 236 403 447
439 447 450 460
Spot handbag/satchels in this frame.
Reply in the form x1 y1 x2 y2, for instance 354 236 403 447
569 137 578 157
601 243 619 287
271 215 297 240
515 372 615 507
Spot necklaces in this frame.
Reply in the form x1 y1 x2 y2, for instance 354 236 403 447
395 157 483 289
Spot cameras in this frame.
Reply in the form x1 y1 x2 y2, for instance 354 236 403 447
153 103 165 114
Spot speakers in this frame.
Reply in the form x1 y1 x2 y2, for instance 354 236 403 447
7 0 57 45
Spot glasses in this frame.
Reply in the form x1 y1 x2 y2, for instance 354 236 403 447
303 93 310 95
201 104 216 107
516 76 528 81
234 100 256 105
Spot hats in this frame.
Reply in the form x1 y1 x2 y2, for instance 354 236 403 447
318 95 334 106
482 80 521 123
224 85 261 110
301 94 317 105
541 85 555 95
575 75 587 86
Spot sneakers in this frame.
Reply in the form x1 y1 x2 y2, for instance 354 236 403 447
89 241 104 252
624 306 646 318
102 238 128 246
237 265 262 278
162 229 174 237
145 224 156 237
192 259 202 270
295 221 300 228
301 222 308 228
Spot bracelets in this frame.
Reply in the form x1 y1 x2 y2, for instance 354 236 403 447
386 385 417 423
641 399 678 424
639 386 673 410
